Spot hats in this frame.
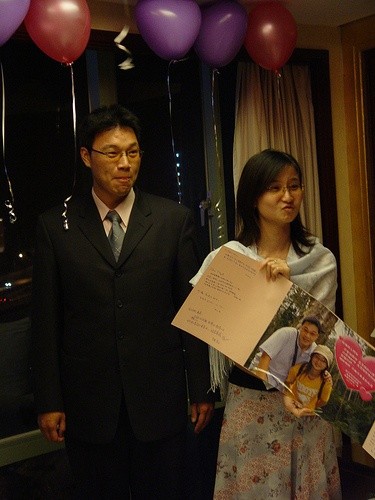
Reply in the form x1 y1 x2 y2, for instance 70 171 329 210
312 345 334 368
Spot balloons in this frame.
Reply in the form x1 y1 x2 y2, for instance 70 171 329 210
193 1 248 71
24 0 91 67
0 0 31 46
135 0 202 63
245 2 297 77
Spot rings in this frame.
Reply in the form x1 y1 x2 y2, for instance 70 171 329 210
274 256 279 264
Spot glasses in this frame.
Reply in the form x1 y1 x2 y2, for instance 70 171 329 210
301 326 319 339
267 180 301 193
313 354 328 364
92 147 142 162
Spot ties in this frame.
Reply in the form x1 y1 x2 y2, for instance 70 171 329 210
107 211 125 262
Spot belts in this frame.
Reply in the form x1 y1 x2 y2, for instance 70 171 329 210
228 366 278 392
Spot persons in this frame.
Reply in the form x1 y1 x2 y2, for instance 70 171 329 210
249 316 333 394
284 344 333 410
23 104 221 500
189 149 342 500
